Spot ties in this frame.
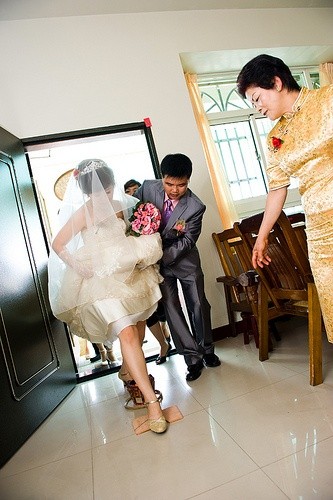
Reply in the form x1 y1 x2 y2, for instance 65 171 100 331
160 200 173 230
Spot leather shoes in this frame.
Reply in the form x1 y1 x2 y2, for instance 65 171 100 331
202 353 221 367
186 359 204 381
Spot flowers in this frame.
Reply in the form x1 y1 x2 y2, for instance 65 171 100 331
271 135 284 153
175 218 189 235
125 201 161 237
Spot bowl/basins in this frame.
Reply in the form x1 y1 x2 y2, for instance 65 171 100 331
123 389 164 418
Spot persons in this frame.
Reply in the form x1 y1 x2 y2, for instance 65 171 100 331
49 160 166 434
123 180 173 364
90 343 119 366
132 154 221 382
237 54 333 344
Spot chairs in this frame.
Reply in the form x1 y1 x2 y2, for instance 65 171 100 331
210 207 324 387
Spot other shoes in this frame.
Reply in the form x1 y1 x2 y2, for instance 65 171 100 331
166 336 170 342
100 360 108 366
156 342 172 364
111 361 118 366
144 399 167 433
90 354 101 363
118 369 130 381
